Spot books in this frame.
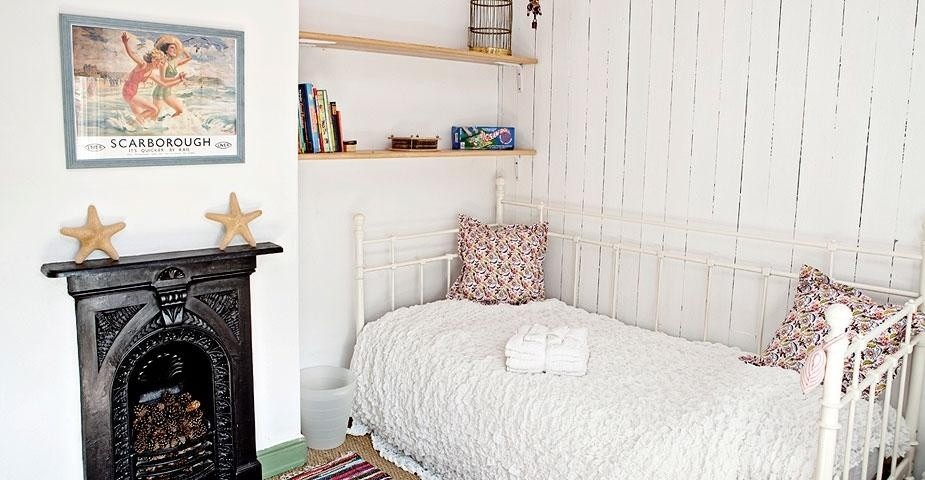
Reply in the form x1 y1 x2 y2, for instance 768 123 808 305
297 81 344 154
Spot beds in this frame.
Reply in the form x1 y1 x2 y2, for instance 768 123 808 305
353 178 925 480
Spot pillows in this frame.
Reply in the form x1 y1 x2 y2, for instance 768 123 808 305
738 265 925 403
446 211 549 305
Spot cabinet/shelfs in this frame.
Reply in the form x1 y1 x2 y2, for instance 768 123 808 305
299 32 538 159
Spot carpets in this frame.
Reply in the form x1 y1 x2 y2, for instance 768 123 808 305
271 451 393 480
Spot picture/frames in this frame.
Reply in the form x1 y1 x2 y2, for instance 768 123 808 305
60 14 245 170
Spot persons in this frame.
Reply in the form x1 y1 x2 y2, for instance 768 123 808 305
120 32 188 132
150 35 192 117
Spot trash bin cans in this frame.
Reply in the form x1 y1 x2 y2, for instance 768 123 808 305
300 365 355 450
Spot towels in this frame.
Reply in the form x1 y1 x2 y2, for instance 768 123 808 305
505 324 590 376
